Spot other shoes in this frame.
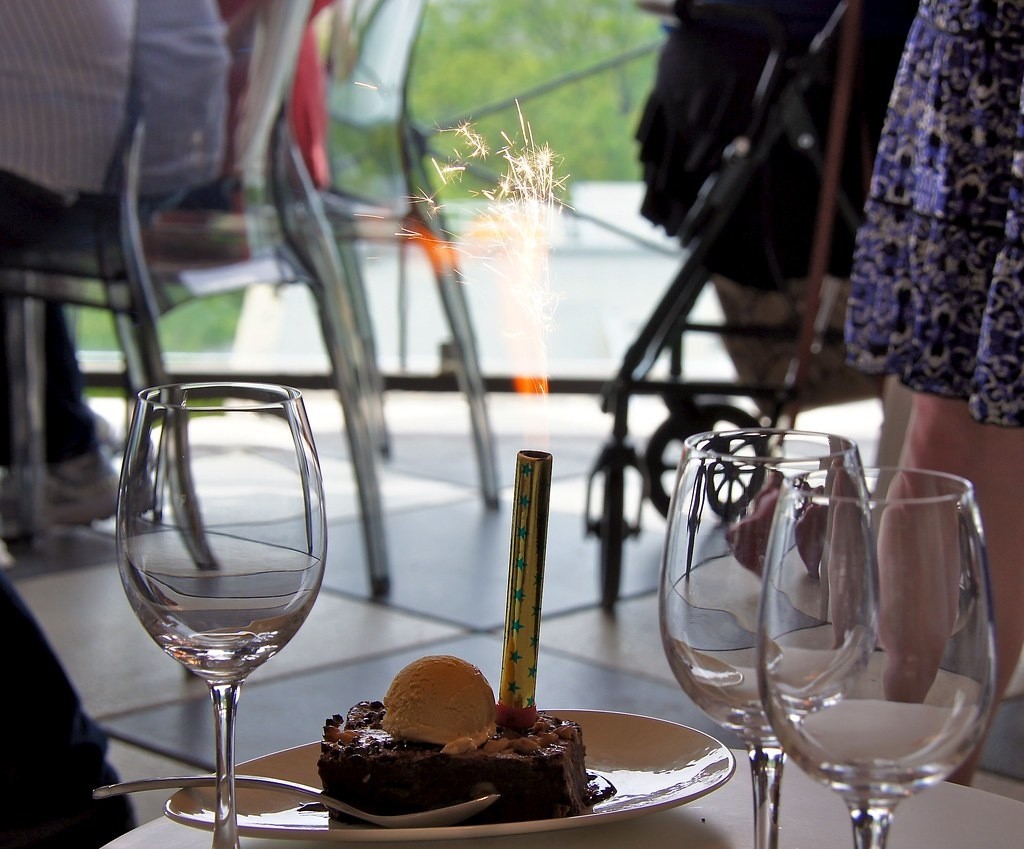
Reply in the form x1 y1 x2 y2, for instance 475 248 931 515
0 451 122 539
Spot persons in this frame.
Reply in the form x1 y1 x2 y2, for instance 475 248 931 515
0 0 235 537
840 0 1024 785
0 571 138 849
183 0 335 209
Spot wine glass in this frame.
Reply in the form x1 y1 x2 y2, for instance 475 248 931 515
756 464 1000 849
113 381 330 849
658 426 876 849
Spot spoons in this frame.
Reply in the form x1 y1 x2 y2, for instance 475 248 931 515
92 774 502 829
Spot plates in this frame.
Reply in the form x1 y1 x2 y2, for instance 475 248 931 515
162 709 737 842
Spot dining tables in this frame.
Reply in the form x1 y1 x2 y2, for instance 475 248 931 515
0 522 1024 849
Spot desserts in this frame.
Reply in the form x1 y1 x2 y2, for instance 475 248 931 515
317 655 589 821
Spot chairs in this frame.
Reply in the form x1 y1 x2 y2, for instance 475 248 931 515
0 0 503 601
